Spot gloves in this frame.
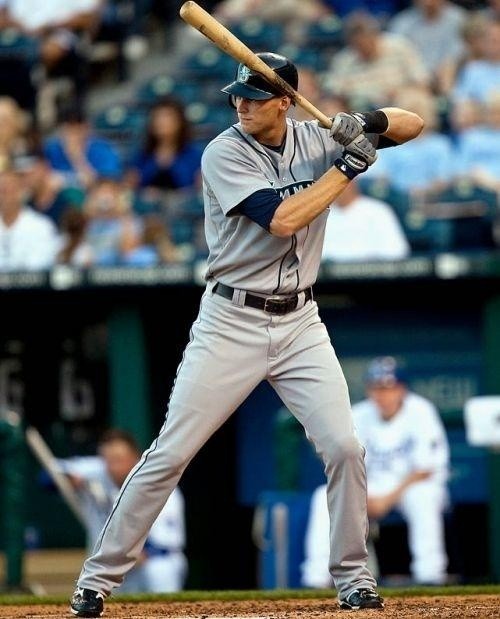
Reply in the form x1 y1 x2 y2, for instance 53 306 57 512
333 130 378 180
317 111 369 146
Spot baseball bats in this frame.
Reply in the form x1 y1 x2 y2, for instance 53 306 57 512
26 427 101 544
179 0 332 127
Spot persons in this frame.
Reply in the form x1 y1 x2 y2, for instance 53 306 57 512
348 356 451 586
66 52 426 617
0 1 500 274
49 429 189 597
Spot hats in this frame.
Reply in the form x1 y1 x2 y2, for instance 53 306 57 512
219 52 298 102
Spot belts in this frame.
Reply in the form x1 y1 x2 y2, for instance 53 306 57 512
212 282 312 316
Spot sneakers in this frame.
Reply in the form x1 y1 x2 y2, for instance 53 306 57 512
70 586 106 617
337 588 384 610
